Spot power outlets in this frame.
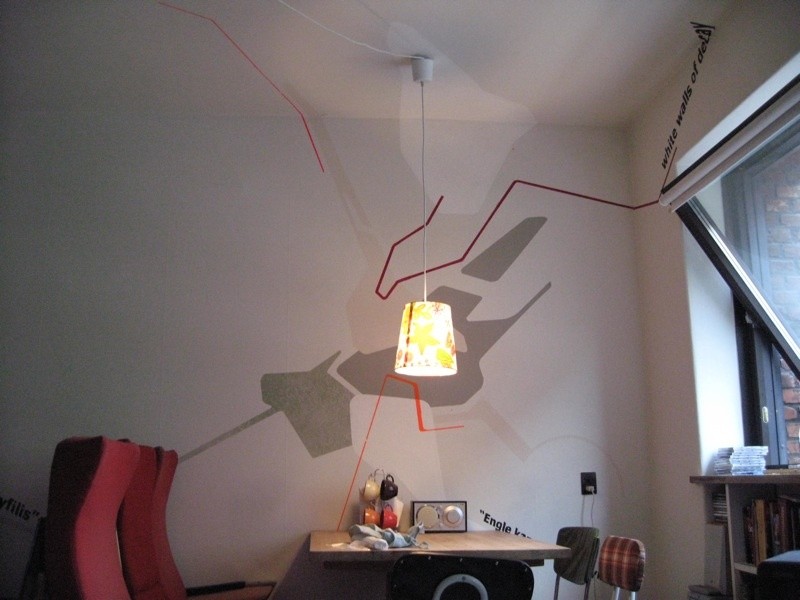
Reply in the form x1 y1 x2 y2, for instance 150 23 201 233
581 472 597 495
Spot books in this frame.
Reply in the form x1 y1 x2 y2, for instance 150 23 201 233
714 446 768 476
712 492 728 524
743 493 800 567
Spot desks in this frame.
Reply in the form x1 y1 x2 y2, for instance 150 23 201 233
309 530 572 600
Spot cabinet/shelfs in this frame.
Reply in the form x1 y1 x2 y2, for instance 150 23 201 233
689 469 800 600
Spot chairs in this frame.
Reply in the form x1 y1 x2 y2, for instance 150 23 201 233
46 436 279 600
598 536 646 600
554 527 599 600
388 554 534 600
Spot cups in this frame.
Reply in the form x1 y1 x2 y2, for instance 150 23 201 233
380 474 398 501
363 475 381 502
363 503 381 525
380 504 398 529
352 505 364 526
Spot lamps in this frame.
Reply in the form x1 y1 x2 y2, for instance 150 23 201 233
394 58 457 376
